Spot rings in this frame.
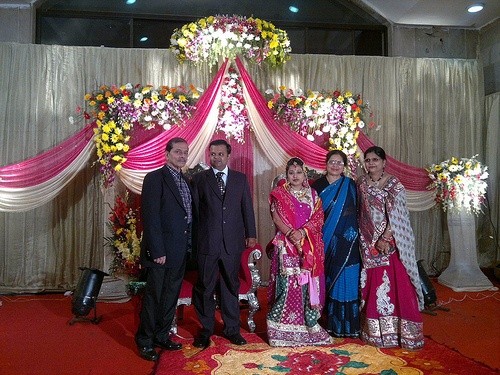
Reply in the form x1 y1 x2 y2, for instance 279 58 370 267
379 249 381 250
381 250 384 251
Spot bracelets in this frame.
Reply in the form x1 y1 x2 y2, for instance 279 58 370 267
299 229 305 236
294 240 300 245
286 229 293 236
382 235 390 242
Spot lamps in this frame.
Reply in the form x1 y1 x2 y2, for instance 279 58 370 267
69 268 104 326
417 259 450 315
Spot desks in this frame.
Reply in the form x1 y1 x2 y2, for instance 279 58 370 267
437 206 499 292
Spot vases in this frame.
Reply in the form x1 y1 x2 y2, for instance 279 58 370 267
128 280 147 295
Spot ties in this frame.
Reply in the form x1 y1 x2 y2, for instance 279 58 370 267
216 172 225 197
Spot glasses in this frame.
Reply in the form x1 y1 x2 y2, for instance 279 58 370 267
327 160 344 165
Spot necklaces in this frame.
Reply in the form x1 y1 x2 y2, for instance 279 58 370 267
370 171 384 186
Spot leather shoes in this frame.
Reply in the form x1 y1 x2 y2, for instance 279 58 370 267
152 338 182 350
192 329 211 347
224 332 247 345
136 343 159 361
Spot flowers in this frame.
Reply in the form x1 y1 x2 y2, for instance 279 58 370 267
103 189 142 276
428 153 489 216
68 9 375 188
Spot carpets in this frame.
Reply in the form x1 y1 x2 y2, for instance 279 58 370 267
153 332 500 375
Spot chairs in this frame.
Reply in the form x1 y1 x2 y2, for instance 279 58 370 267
176 244 262 331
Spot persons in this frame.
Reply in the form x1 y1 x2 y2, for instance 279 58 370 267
134 137 196 360
312 150 361 337
267 158 334 346
356 145 423 348
190 139 257 347
